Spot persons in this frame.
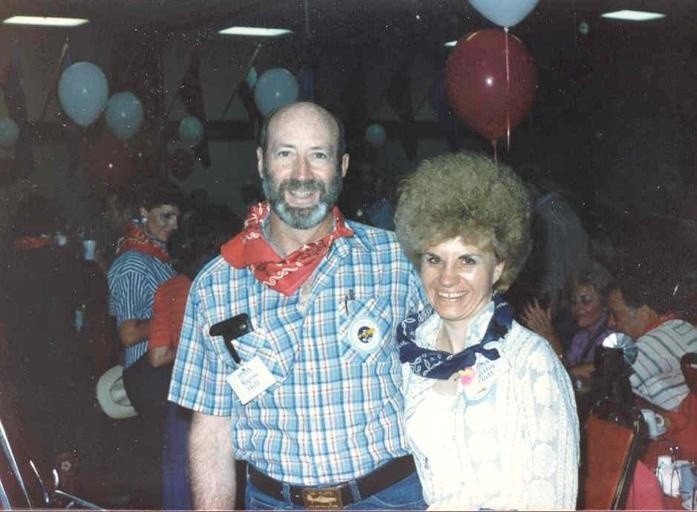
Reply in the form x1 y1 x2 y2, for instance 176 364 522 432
1 98 695 511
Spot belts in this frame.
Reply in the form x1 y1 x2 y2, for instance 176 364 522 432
248 455 415 510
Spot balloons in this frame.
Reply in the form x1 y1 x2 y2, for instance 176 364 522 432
445 27 538 142
469 0 539 27
103 89 146 143
254 66 301 118
58 60 110 131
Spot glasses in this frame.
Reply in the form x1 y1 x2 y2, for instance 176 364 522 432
570 294 593 304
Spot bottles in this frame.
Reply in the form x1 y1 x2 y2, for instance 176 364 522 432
64 304 89 385
38 232 66 247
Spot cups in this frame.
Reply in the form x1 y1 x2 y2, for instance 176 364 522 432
82 240 96 261
641 409 664 435
656 455 696 510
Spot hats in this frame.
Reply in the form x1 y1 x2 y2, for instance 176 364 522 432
96 366 138 419
